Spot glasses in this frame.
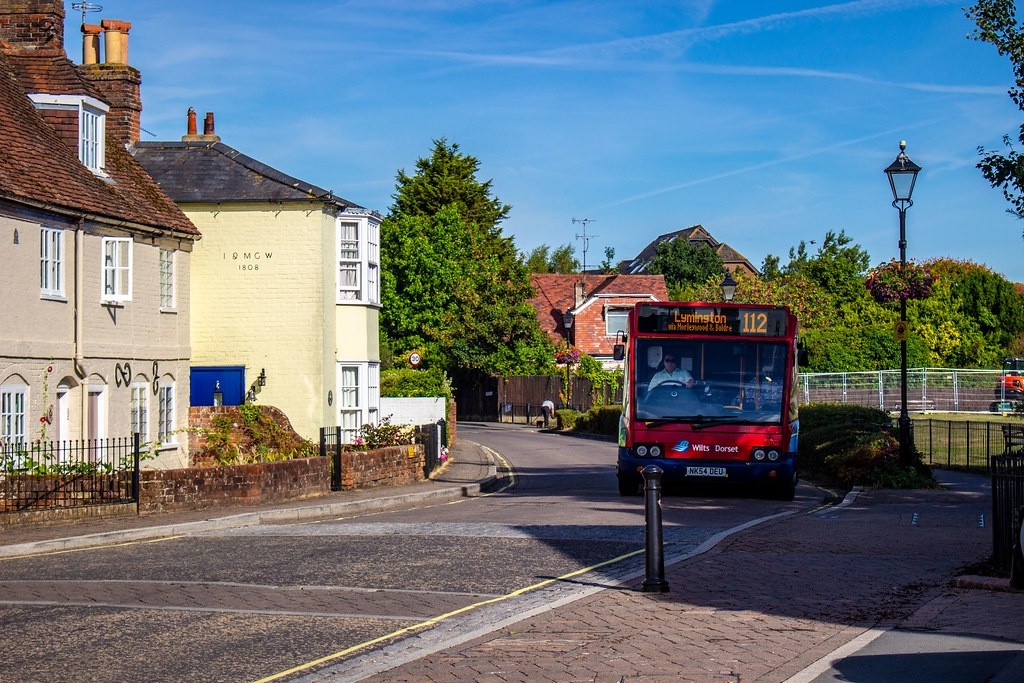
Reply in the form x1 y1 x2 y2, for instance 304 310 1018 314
663 358 677 363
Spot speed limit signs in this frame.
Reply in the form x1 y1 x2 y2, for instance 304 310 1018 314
409 352 421 367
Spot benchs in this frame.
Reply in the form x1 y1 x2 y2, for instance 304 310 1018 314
895 400 937 410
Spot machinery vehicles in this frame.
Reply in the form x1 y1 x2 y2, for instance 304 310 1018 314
989 359 1024 418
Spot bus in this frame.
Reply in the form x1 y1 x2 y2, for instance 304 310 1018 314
613 300 809 501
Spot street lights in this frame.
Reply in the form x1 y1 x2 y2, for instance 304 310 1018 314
882 138 922 475
560 309 574 412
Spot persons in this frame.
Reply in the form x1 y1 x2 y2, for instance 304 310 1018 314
647 349 695 394
541 400 554 428
731 363 783 406
